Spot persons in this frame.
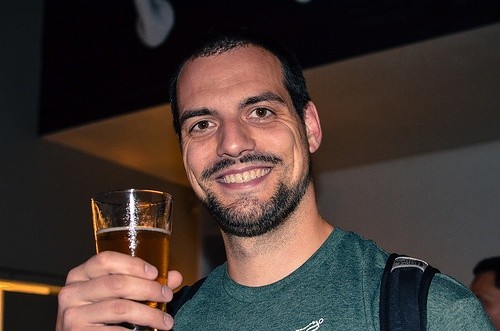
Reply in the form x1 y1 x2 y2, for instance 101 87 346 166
56 37 500 331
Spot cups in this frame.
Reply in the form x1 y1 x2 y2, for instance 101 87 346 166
92 189 174 331
